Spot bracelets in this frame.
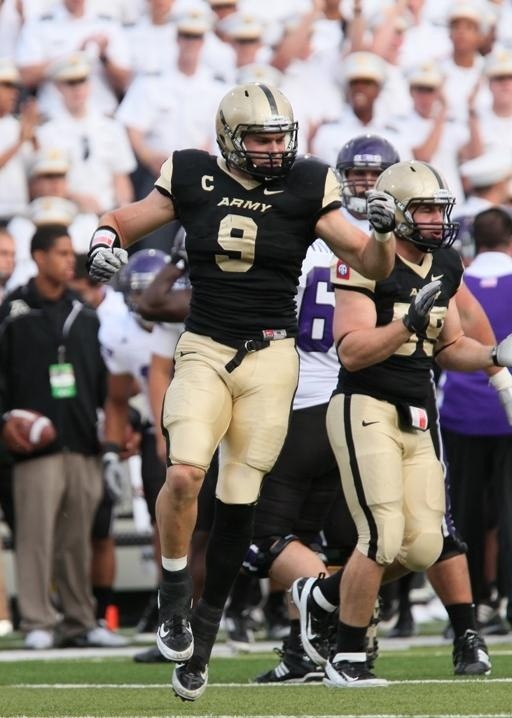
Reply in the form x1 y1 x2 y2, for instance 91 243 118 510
402 319 416 334
490 342 498 366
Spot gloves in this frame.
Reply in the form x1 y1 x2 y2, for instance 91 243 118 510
495 331 512 368
363 188 396 238
86 246 129 283
407 280 444 335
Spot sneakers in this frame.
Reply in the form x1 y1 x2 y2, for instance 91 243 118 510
73 625 127 650
170 609 221 703
25 630 54 651
385 584 509 639
134 641 175 668
324 648 387 688
361 594 383 668
287 574 337 668
450 628 491 678
248 645 319 687
153 577 196 662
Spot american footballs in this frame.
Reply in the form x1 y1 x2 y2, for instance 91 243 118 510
6 408 57 448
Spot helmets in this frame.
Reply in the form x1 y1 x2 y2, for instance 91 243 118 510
335 135 401 180
215 83 297 178
115 248 189 303
373 160 454 245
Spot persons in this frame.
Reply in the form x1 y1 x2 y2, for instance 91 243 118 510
87 82 396 701
289 160 511 686
0 0 512 664
284 135 512 676
243 214 380 682
